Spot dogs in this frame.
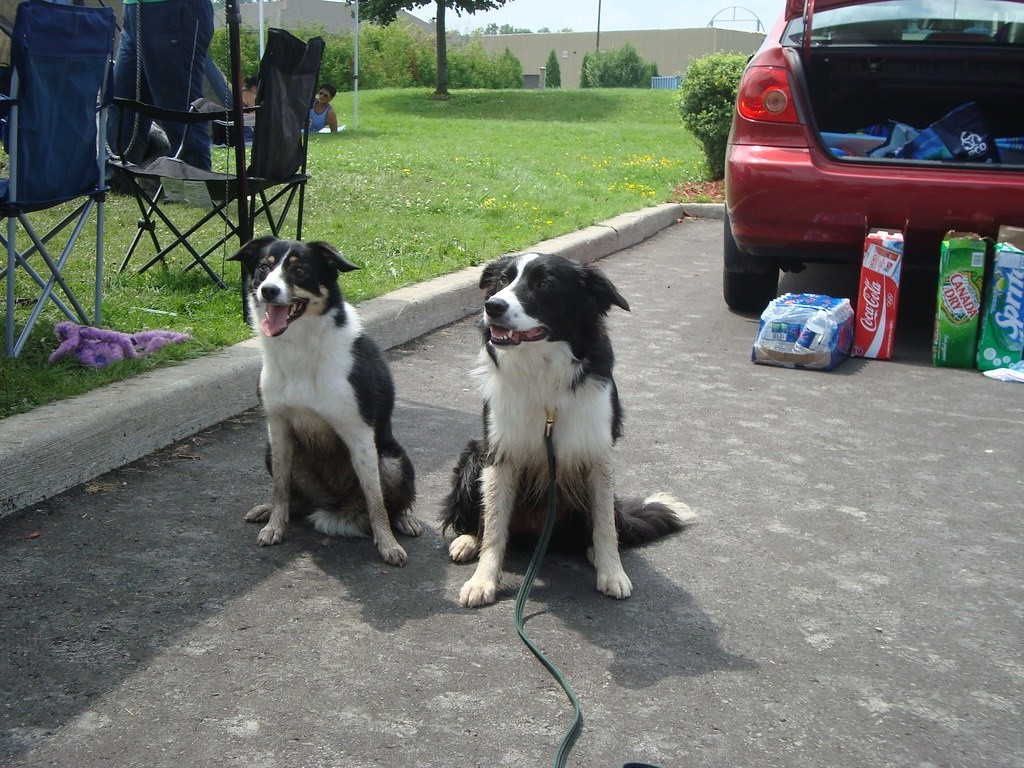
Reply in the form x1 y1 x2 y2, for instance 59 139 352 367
223 234 700 610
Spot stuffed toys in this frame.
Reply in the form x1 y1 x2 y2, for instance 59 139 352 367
47 320 188 367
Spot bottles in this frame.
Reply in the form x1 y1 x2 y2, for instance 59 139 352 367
762 293 854 354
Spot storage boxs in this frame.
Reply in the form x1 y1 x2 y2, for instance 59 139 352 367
851 216 911 359
932 230 986 369
974 225 1024 371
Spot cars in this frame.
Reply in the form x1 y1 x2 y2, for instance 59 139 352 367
723 0 1024 315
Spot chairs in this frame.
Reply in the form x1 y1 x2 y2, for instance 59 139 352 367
125 26 325 286
97 0 266 290
0 0 117 362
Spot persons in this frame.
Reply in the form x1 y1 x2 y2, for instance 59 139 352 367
309 82 339 134
240 74 259 114
